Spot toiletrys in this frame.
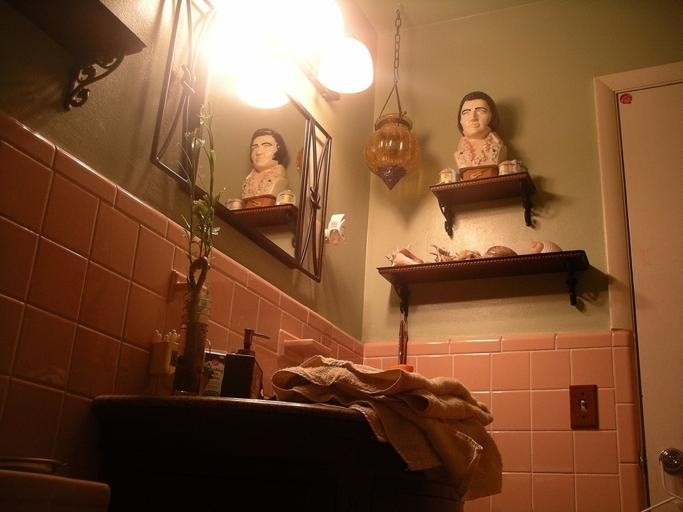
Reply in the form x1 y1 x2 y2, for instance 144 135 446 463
218 328 270 400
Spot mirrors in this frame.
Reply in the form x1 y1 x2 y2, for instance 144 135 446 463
150 0 332 283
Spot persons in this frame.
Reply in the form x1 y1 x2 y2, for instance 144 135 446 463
453 91 508 169
241 128 288 198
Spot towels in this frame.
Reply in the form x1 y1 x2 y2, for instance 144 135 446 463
269 354 494 504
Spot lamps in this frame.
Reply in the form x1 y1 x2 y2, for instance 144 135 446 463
174 101 219 396
364 9 414 191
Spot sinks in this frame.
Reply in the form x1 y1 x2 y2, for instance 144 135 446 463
89 394 428 511
